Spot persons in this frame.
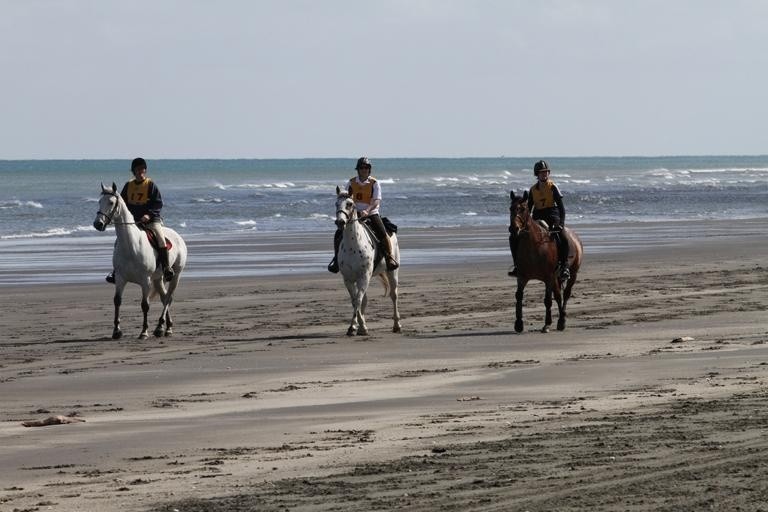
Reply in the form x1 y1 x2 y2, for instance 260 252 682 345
508 160 571 281
328 157 396 271
106 159 174 281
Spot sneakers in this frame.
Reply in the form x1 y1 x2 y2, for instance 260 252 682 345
508 267 517 276
561 269 571 280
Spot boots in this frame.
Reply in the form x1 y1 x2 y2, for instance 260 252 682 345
329 236 343 273
381 232 398 269
159 247 174 281
106 270 115 283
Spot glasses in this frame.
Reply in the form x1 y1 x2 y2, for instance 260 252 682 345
359 166 370 170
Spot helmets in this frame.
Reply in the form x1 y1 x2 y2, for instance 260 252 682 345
534 160 550 176
132 158 146 169
357 158 371 168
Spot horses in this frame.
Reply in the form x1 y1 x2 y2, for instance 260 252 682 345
92 181 189 341
335 185 403 336
509 189 584 333
